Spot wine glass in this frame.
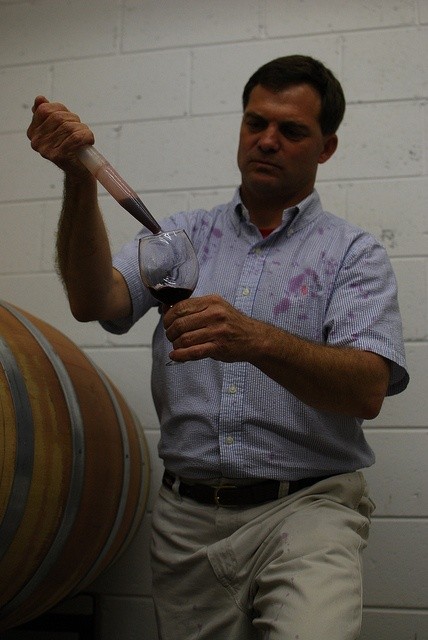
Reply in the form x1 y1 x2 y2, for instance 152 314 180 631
137 229 201 367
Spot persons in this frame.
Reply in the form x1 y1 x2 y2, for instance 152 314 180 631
26 55 409 636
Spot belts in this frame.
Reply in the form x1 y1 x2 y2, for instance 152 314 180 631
162 470 321 507
